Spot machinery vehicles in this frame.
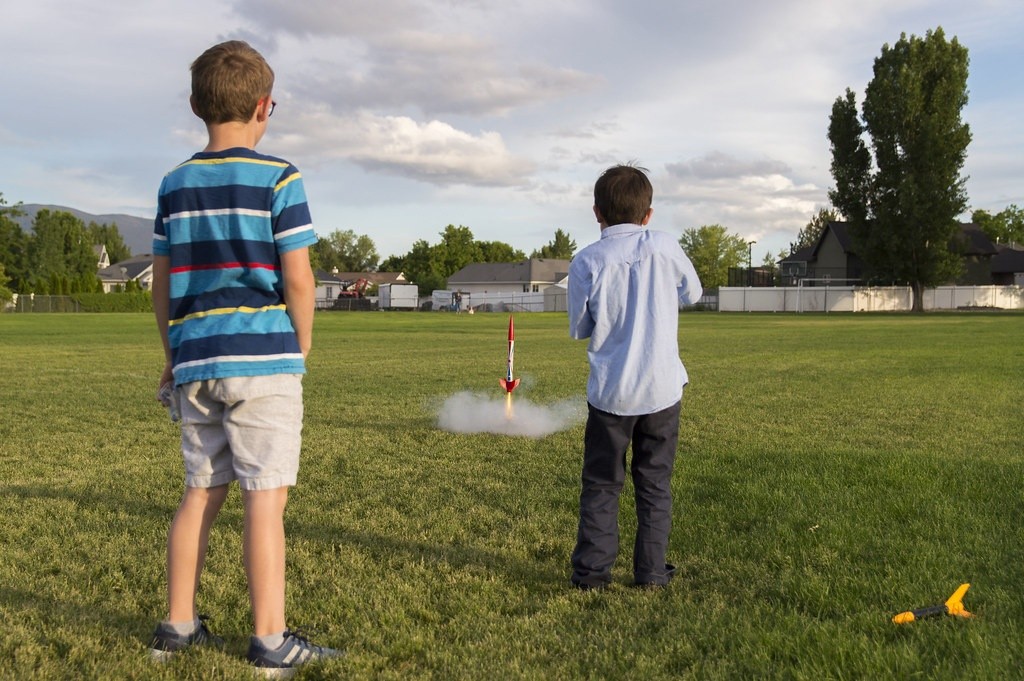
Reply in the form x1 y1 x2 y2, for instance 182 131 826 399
336 278 368 310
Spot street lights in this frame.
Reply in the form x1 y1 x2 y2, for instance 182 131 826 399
748 240 757 287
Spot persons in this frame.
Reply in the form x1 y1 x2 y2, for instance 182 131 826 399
455 293 462 315
567 156 703 591
148 40 345 680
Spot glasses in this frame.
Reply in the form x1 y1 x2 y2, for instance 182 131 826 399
257 100 276 117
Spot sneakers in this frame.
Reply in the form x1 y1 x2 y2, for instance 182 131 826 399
147 614 225 663
245 628 348 680
